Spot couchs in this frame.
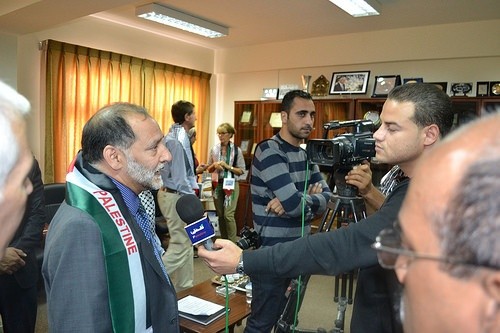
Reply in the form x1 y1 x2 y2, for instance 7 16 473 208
151 186 170 249
36 182 68 305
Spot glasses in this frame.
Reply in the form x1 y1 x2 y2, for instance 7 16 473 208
216 132 229 136
370 228 488 269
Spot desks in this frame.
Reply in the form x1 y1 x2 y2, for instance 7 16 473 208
173 269 299 333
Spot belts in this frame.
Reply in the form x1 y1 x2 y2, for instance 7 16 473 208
161 187 177 194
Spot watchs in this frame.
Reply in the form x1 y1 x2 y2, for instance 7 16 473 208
236 251 247 276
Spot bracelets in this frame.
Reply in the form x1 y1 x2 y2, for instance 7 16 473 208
230 166 234 171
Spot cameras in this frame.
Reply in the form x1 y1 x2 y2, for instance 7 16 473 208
307 120 375 167
236 224 262 250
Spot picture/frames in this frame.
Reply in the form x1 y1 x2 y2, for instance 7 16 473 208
489 81 500 96
372 74 400 97
425 81 448 93
329 70 371 94
475 81 489 97
403 78 423 86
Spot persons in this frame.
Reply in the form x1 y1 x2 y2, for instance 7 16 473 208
206 122 244 245
244 90 331 333
152 100 202 292
0 79 35 266
347 161 406 218
0 155 42 333
200 81 454 333
372 107 499 332
44 101 183 333
185 125 209 258
333 75 347 91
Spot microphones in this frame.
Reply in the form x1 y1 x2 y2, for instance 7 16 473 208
176 194 226 276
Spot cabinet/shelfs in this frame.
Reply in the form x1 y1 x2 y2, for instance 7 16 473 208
232 95 500 236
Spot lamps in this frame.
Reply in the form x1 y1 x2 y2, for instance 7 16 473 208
135 3 229 40
331 1 384 17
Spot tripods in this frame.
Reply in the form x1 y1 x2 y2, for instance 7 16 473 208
273 167 369 333
